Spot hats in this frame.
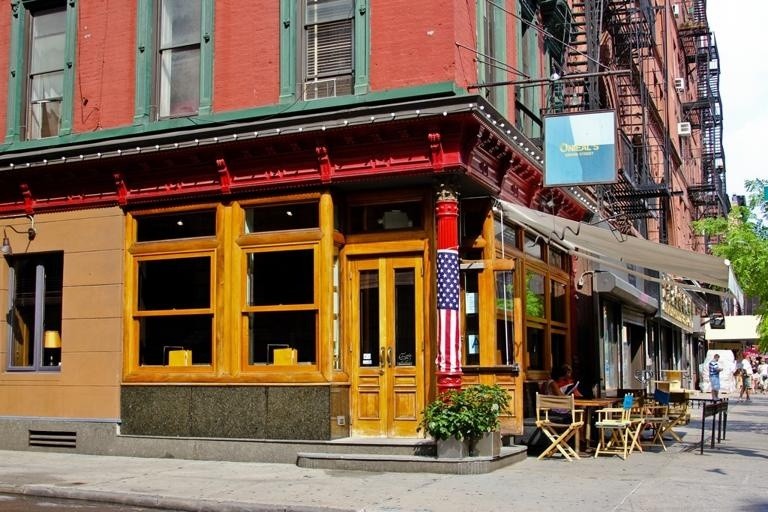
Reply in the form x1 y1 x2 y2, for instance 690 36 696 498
742 353 748 358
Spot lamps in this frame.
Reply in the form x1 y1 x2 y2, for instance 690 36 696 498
44 330 61 366
548 57 564 81
1 215 37 255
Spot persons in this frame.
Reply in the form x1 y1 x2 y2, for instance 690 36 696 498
545 363 584 425
709 354 767 402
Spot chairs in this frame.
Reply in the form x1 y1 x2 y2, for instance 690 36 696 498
535 388 689 462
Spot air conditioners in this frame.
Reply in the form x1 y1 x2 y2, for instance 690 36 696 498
677 122 691 137
672 4 679 18
675 78 685 95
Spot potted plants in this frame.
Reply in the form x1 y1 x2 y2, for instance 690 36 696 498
417 384 512 458
